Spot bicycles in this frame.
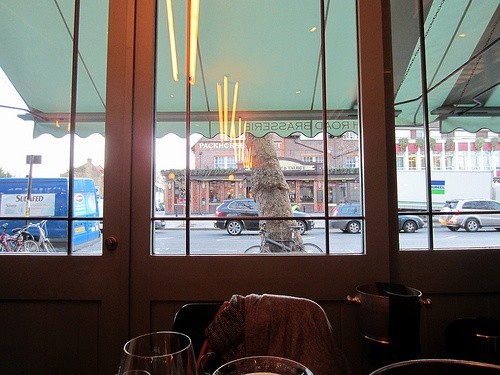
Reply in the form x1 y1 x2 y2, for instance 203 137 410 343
0 221 56 252
242 222 324 253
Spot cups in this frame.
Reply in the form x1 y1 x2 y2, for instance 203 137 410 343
123 370 151 375
118 331 198 375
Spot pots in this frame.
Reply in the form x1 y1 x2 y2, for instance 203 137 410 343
341 281 433 338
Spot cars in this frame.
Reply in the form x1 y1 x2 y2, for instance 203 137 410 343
438 200 500 232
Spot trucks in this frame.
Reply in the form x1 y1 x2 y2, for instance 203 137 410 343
0 178 102 254
396 169 500 222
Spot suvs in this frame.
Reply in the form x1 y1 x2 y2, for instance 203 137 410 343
326 205 426 235
212 198 316 236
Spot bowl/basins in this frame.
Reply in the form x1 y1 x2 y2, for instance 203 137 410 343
212 356 313 375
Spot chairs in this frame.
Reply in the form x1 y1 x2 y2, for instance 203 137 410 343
196 293 346 375
170 302 224 375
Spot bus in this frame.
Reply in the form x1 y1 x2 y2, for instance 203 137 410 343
155 184 167 232
